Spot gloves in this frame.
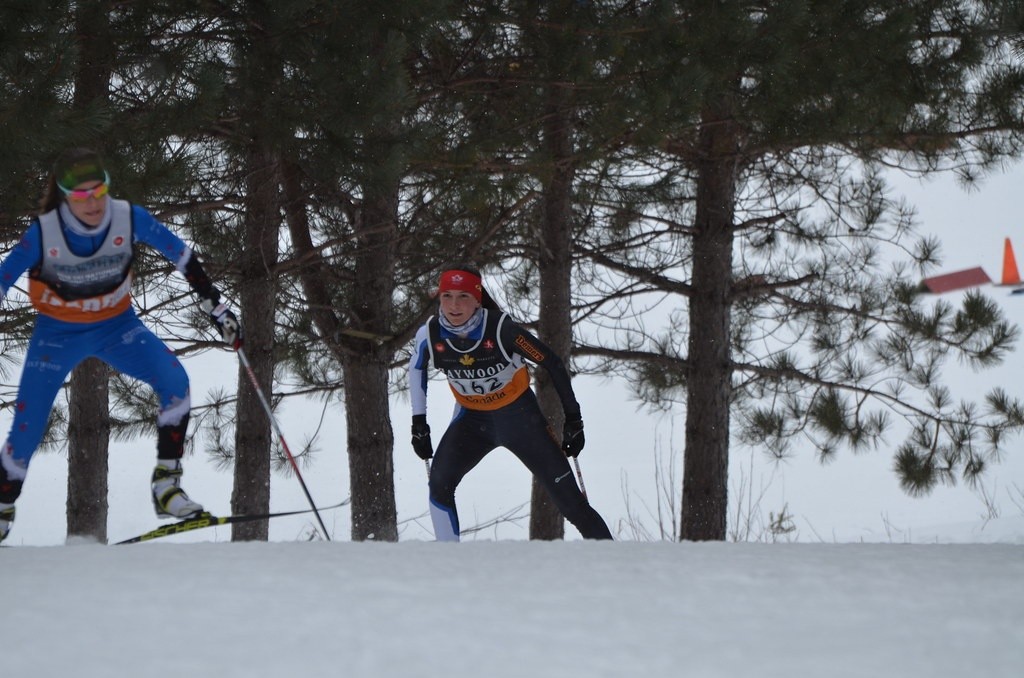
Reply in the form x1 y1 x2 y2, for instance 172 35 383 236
562 420 585 458
412 424 433 459
210 304 241 351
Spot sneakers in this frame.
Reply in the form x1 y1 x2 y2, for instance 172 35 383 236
151 485 203 519
0 519 17 542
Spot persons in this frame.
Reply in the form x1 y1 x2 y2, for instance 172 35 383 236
0 149 243 541
408 264 612 541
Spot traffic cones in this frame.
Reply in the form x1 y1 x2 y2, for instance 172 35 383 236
994 238 1024 286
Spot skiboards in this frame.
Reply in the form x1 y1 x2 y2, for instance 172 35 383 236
111 496 351 547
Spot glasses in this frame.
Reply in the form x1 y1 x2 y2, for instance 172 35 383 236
68 182 108 201
